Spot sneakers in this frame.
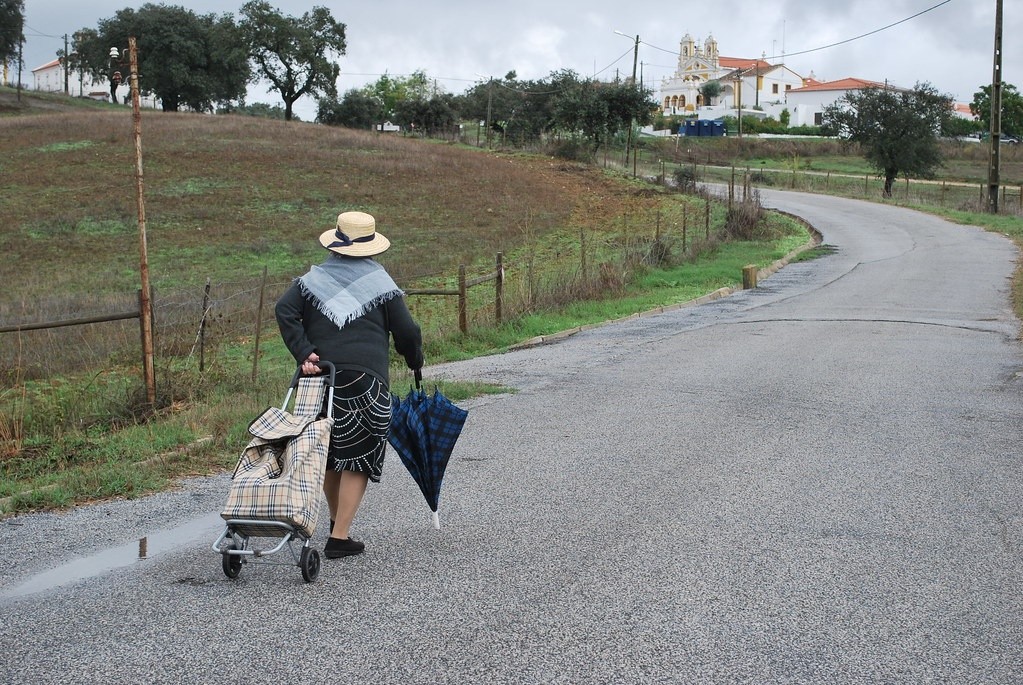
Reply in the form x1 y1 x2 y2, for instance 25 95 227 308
324 537 365 558
329 519 334 534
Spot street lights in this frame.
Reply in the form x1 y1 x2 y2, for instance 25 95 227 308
614 30 638 81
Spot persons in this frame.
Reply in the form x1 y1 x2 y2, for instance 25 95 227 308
275 211 423 559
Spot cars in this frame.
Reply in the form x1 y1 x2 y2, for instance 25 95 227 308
980 132 1019 145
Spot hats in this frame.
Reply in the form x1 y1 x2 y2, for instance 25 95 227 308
319 211 391 258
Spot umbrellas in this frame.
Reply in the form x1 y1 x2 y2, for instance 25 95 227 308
387 368 469 529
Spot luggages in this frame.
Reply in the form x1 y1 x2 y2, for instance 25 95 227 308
219 359 336 538
